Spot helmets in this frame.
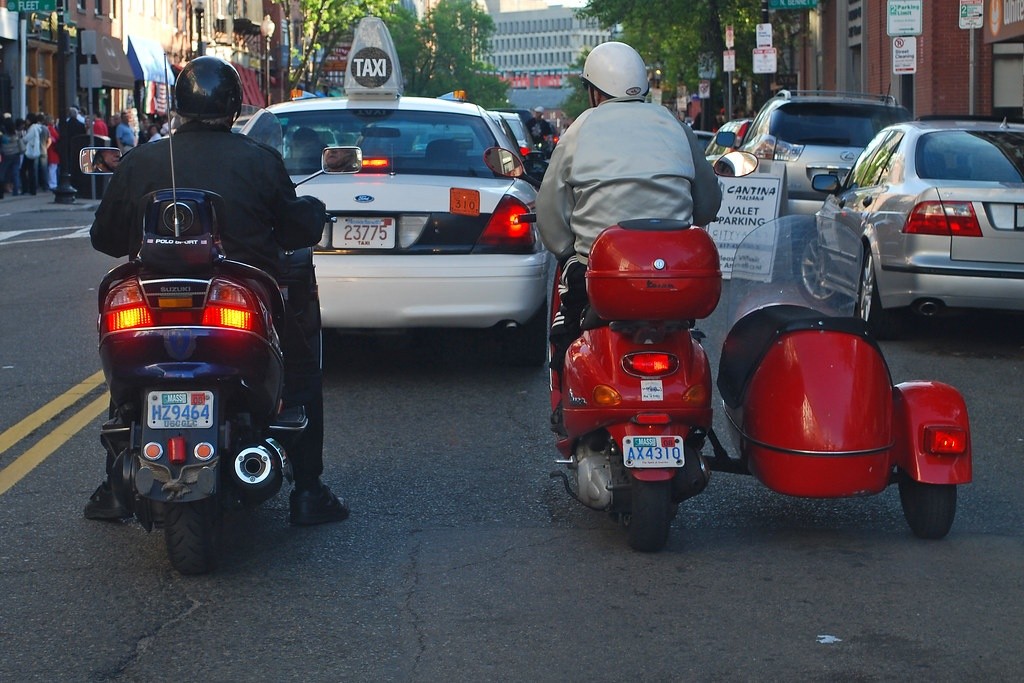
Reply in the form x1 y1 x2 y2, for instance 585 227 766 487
174 56 244 117
720 156 736 176
583 42 649 99
90 149 100 166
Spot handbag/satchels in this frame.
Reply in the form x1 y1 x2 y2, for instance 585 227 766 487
17 138 26 154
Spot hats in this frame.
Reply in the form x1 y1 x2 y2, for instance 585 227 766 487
534 106 545 114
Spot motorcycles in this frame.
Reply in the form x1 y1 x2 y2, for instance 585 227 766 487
481 146 972 555
73 139 367 575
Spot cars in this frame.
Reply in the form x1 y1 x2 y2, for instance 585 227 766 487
249 15 553 367
793 117 1024 336
489 107 549 184
703 118 754 172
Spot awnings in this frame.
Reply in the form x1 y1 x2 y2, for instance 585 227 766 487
79 30 264 108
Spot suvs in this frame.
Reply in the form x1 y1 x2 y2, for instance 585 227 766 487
712 87 921 271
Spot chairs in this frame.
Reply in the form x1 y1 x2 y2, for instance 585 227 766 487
424 138 471 175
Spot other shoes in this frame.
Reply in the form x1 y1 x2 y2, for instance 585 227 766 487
549 400 565 434
84 481 133 519
289 484 350 524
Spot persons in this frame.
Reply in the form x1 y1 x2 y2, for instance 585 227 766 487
290 127 321 159
0 106 180 201
91 149 119 172
324 149 356 172
526 42 761 436
84 57 348 526
